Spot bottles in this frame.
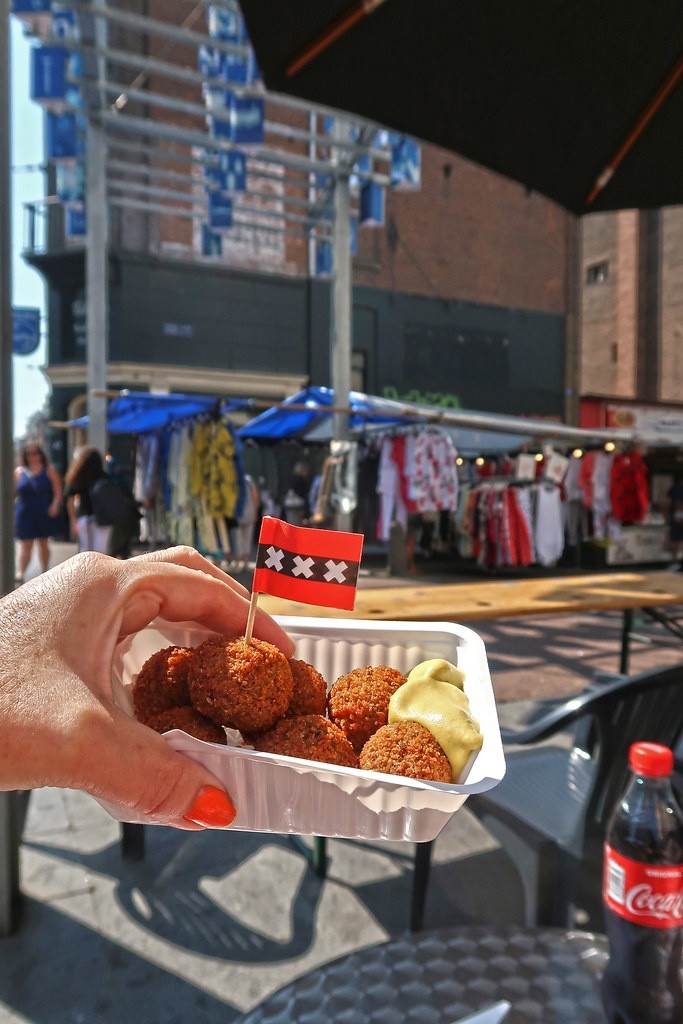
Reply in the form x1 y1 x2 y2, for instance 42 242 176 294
600 741 683 1024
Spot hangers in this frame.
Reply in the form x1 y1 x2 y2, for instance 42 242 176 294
390 418 442 436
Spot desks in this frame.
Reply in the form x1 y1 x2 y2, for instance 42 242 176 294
243 572 683 874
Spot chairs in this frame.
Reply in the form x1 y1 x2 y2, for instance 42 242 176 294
406 661 683 934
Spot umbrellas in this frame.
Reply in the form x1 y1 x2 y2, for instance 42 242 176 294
234 0 683 218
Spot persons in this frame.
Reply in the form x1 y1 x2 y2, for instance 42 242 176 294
15 442 63 586
63 447 113 556
0 545 298 832
